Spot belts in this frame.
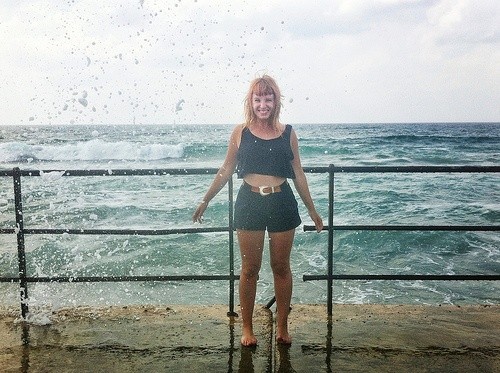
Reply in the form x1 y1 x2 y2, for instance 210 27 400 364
250 185 281 195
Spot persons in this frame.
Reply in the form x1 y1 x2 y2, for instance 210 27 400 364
191 73 324 347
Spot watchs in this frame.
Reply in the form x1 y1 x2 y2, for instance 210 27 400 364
201 201 209 206
